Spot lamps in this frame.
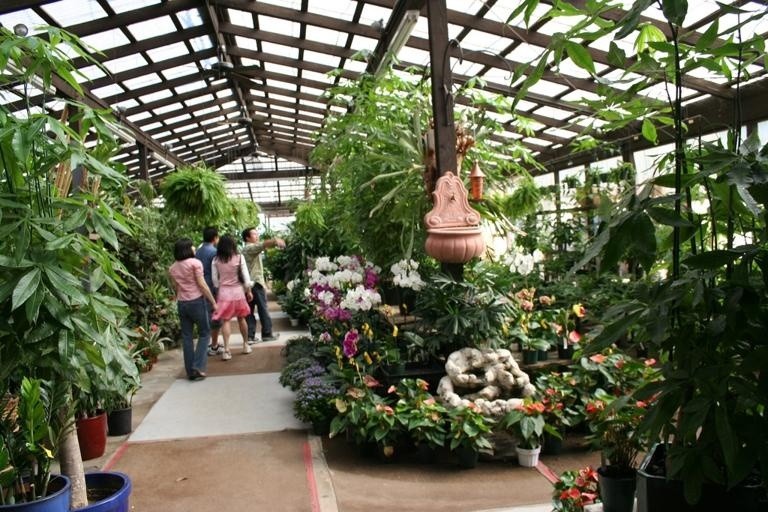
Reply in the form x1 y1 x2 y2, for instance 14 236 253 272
469 158 486 200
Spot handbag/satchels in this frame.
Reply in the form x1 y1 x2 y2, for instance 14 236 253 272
238 254 245 284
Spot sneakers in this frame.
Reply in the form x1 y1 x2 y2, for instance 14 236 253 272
222 351 232 360
248 337 259 345
263 336 276 341
207 345 224 357
242 343 253 354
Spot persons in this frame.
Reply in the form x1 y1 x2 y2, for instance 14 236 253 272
241 227 285 344
211 234 253 360
196 227 225 356
169 238 219 380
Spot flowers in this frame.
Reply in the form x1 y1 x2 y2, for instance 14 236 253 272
570 351 662 469
329 387 400 442
499 403 551 448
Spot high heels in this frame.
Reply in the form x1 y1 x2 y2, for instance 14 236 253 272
188 368 206 380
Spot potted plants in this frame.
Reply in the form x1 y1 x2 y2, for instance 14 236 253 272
107 346 141 436
293 377 339 436
0 22 144 512
575 142 768 512
72 395 107 459
0 379 70 512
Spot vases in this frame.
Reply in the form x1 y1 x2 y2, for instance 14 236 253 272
515 443 542 467
596 464 637 512
359 439 399 466
